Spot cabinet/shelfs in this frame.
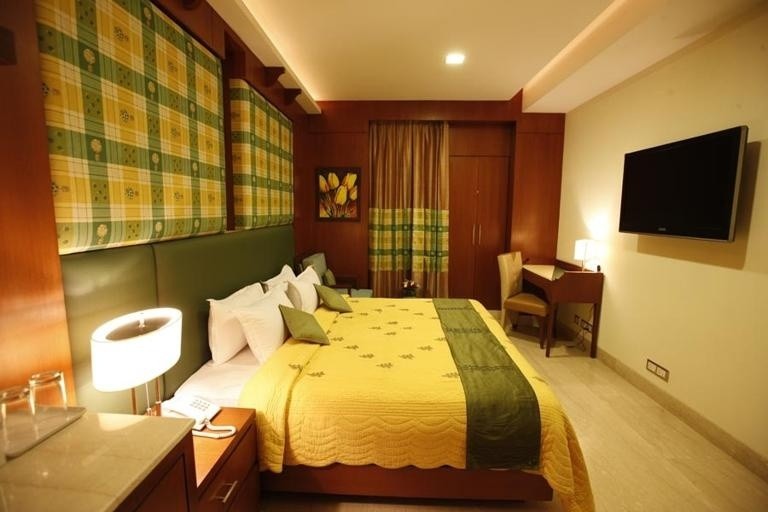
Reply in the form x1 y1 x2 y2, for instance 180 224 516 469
448 123 512 310
0 406 199 510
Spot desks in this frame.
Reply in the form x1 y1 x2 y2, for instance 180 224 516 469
517 253 607 360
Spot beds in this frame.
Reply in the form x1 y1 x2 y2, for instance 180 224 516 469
166 289 598 511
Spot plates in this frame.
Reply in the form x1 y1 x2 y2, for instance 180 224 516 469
0 405 84 459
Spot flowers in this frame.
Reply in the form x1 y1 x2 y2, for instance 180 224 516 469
318 172 359 219
396 276 419 296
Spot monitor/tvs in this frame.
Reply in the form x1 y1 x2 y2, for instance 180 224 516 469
619 125 749 242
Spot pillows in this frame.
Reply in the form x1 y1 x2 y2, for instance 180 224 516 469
229 287 298 365
321 266 337 287
314 279 355 315
202 279 265 367
280 263 323 316
277 303 332 347
261 264 299 291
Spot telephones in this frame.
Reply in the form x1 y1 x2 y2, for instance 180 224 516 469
160 393 222 431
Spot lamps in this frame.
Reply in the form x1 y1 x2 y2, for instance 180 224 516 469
86 302 184 415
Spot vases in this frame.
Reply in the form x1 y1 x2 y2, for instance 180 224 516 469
401 287 417 298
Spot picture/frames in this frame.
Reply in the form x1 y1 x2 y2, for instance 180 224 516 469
312 164 362 221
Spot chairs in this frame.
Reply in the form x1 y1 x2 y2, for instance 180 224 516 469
298 251 377 298
496 247 562 351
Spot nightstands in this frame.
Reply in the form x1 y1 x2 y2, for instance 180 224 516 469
140 402 268 512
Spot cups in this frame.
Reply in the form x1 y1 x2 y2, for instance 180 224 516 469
27 369 68 428
0 385 38 449
